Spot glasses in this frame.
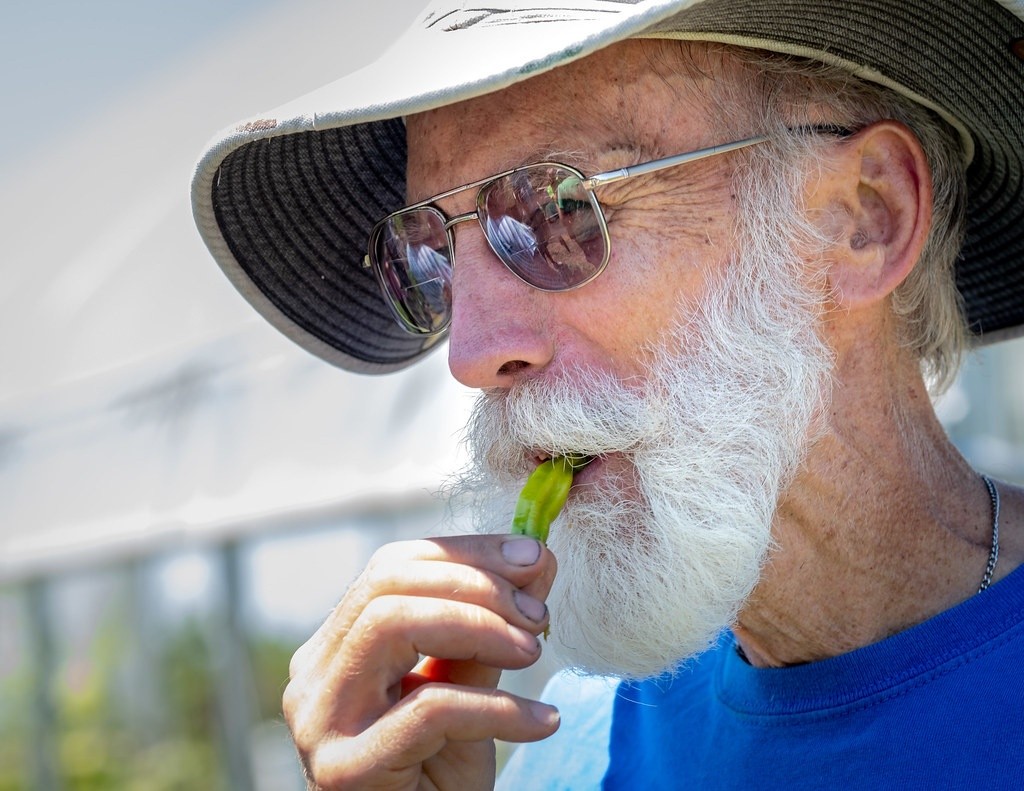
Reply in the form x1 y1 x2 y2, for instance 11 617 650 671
360 123 861 338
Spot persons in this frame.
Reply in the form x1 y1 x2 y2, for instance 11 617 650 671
399 213 452 321
483 167 604 289
282 0 1024 791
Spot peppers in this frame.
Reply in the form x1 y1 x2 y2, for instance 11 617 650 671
508 452 585 548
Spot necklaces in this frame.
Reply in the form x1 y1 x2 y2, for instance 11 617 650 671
976 474 1000 594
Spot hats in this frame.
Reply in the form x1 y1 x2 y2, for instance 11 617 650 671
191 0 1024 374
